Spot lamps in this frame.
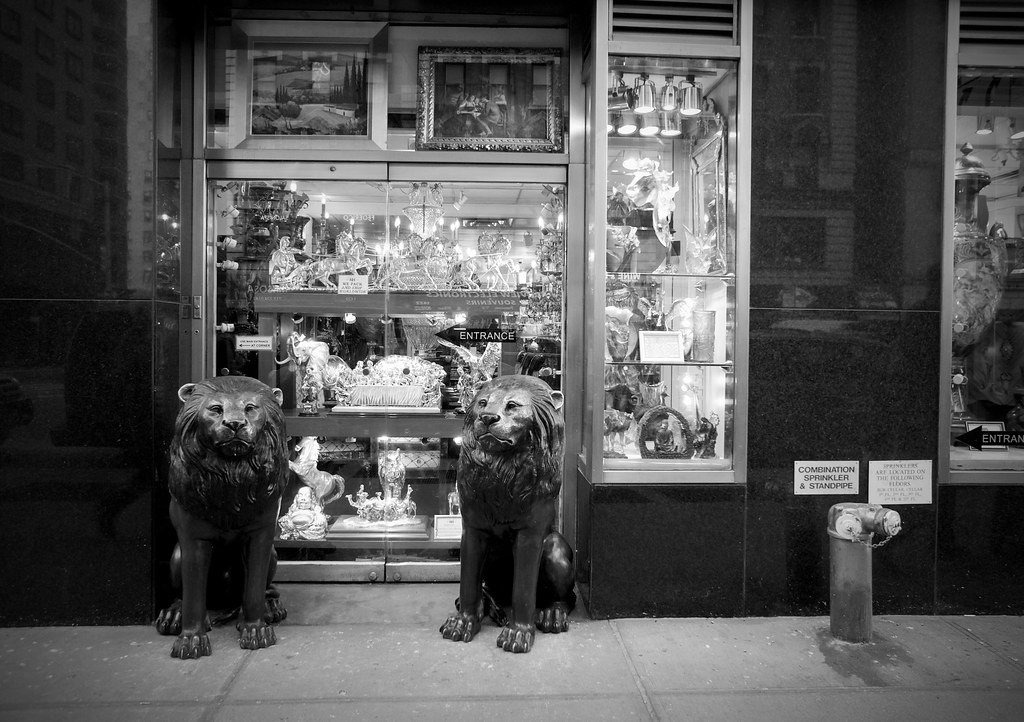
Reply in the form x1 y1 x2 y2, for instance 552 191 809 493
660 113 682 137
217 236 237 249
222 204 240 218
452 190 468 212
1008 119 1024 140
677 76 704 116
631 74 657 114
608 114 615 135
617 113 638 136
321 193 327 219
975 114 995 135
541 223 558 239
660 74 679 114
217 260 239 271
541 204 557 217
638 114 660 137
608 71 633 112
217 181 241 198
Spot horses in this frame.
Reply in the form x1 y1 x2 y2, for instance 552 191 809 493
305 230 518 292
288 435 344 506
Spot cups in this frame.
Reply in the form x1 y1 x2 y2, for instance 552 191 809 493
692 310 715 362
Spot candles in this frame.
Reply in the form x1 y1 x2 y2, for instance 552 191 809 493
394 217 401 237
451 223 456 242
350 218 356 237
455 220 460 242
375 243 380 265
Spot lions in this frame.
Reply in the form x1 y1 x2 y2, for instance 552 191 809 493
439 374 577 655
156 376 288 661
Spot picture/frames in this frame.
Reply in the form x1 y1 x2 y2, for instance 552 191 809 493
229 19 387 154
411 44 565 153
638 331 684 363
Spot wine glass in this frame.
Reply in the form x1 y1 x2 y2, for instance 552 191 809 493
603 409 632 458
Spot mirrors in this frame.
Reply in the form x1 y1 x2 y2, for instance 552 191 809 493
327 213 542 288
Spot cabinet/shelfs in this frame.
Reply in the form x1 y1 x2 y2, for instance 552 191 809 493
254 289 522 549
601 137 735 473
